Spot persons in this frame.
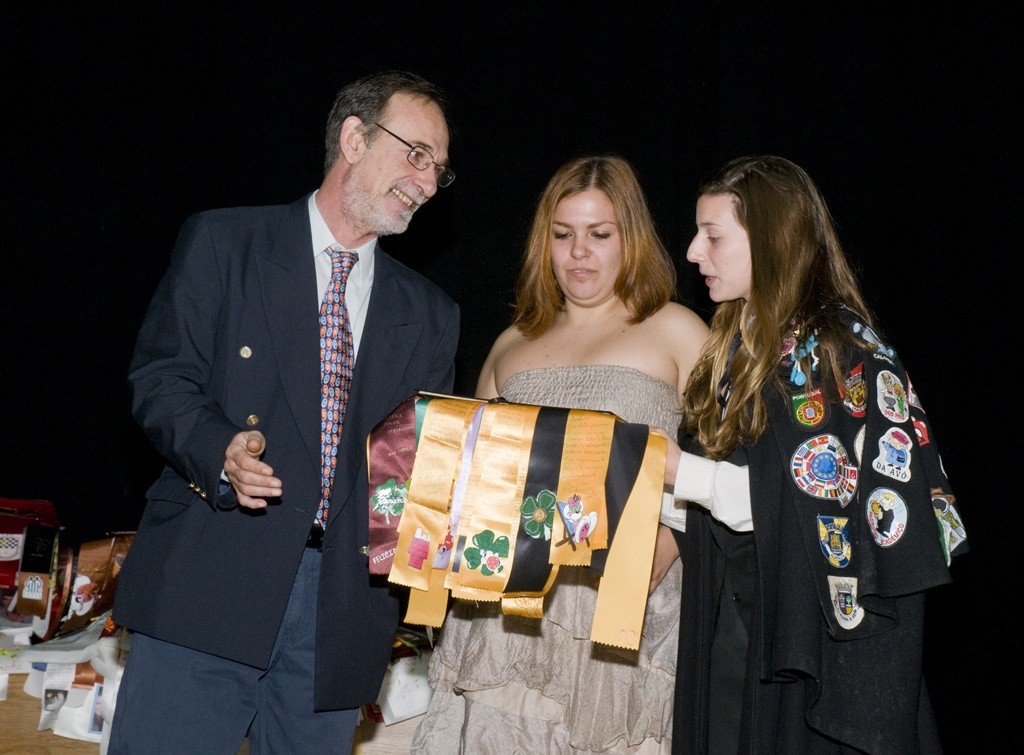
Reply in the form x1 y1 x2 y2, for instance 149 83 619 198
107 70 461 755
407 156 712 755
651 155 970 755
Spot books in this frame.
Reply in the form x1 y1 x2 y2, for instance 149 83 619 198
418 391 628 424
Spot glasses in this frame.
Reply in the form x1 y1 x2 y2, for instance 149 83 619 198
375 121 456 188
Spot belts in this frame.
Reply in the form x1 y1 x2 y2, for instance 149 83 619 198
304 527 325 552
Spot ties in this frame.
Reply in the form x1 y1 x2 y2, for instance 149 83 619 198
313 247 360 530
